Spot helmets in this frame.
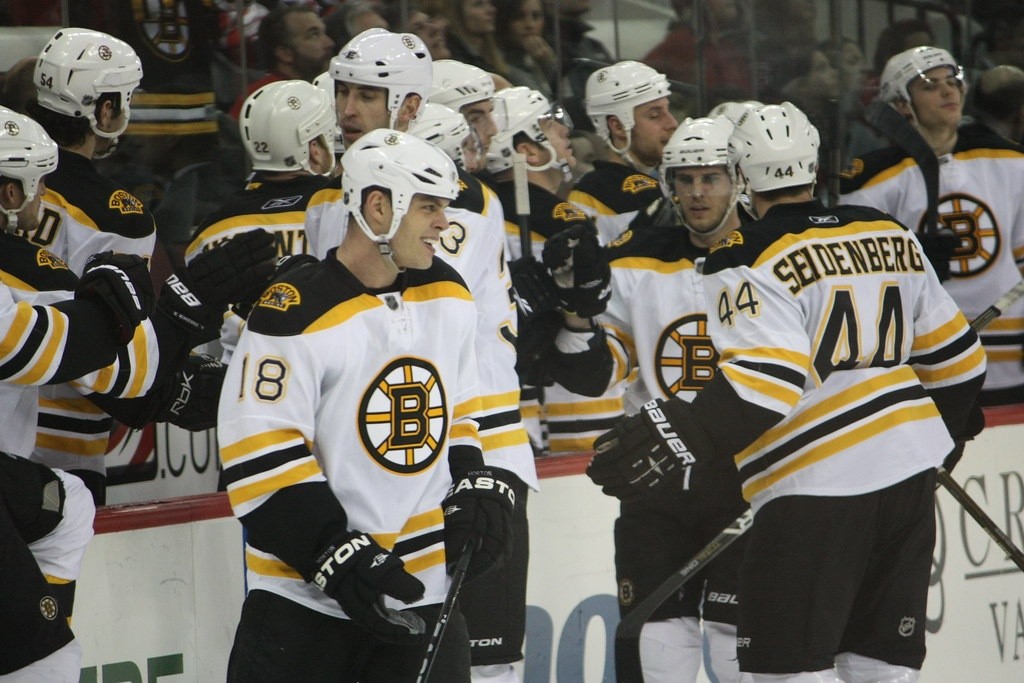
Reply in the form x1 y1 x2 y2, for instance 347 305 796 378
706 101 766 122
733 102 821 192
404 103 469 172
239 80 337 176
585 61 671 154
33 28 143 138
659 116 735 199
0 104 58 199
484 85 556 173
881 45 956 105
329 28 433 128
341 128 459 241
431 57 495 113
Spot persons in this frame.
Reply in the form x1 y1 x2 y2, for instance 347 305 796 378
0 0 678 504
585 101 991 683
831 47 1024 407
0 103 158 683
633 0 1024 217
218 128 519 682
539 114 761 682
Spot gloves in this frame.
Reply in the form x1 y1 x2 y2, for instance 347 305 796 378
444 466 514 584
509 255 565 358
162 228 276 335
152 353 228 432
76 251 155 348
543 224 612 317
311 529 427 647
587 399 715 507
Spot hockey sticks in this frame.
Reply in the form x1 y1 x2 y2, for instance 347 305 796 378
413 532 474 683
935 465 1024 570
514 152 532 258
826 96 843 211
864 98 938 233
615 281 1023 683
